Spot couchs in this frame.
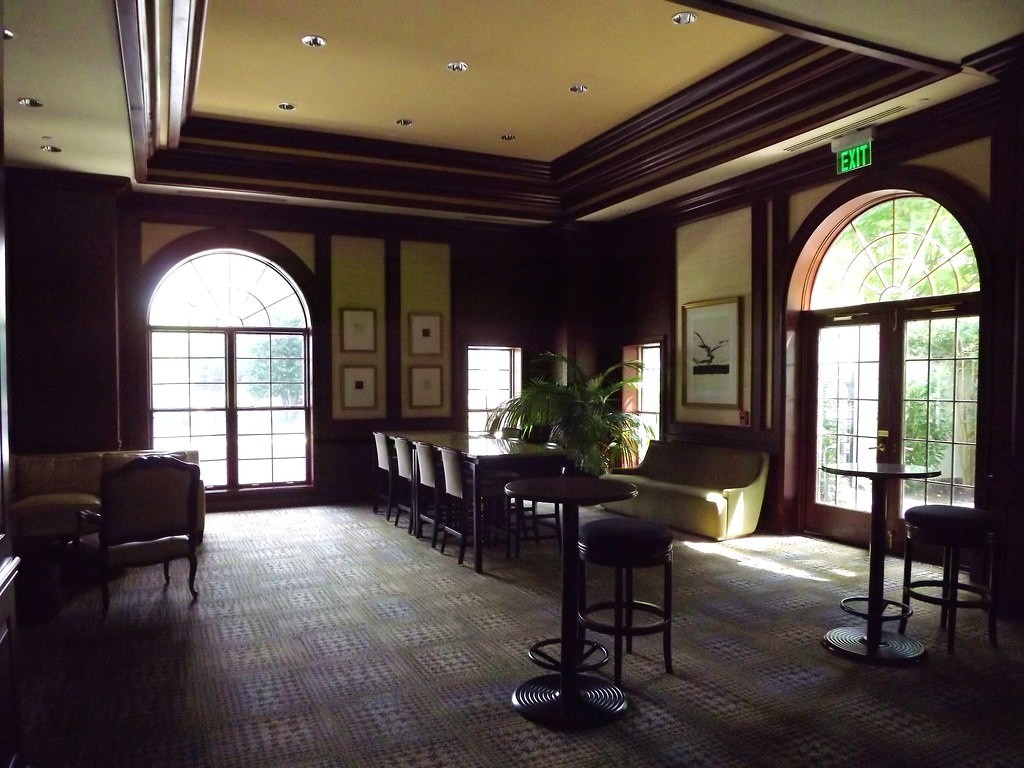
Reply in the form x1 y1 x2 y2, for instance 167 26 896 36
600 440 771 543
7 448 206 548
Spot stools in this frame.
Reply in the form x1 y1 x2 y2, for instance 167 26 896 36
577 517 675 685
898 504 1000 651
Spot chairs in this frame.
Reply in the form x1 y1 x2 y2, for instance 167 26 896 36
372 431 561 565
64 456 201 612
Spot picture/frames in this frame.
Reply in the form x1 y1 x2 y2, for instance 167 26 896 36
682 295 744 410
407 364 444 409
338 364 379 411
407 310 444 358
338 307 377 354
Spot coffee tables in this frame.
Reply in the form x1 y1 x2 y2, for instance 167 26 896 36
822 462 941 661
504 476 639 727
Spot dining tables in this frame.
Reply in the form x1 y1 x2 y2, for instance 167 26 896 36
380 428 567 575
